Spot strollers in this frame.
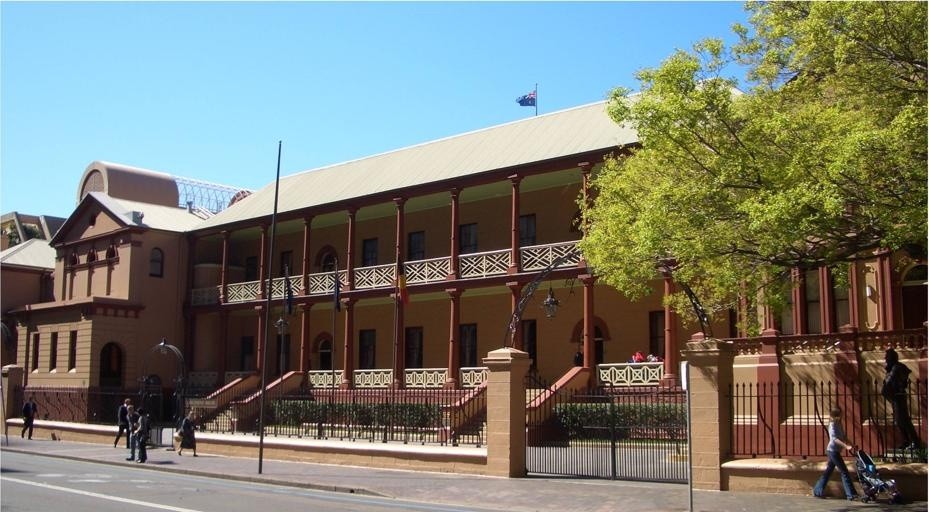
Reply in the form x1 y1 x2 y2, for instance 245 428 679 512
846 444 904 505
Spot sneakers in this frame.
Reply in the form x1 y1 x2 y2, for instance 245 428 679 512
193 454 198 457
811 489 825 499
127 454 145 464
848 493 861 502
176 450 181 455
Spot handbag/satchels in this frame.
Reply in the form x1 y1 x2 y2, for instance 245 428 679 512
174 429 184 441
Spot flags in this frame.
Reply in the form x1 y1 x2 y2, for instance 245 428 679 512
513 89 535 108
285 276 294 318
396 250 409 305
334 274 342 313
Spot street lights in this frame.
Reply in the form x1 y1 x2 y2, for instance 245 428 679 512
273 317 289 400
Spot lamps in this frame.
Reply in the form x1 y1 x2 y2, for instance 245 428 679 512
274 311 288 335
543 281 559 321
866 285 872 297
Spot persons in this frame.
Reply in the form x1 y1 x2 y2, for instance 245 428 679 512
176 410 199 456
879 346 925 452
21 393 38 440
573 345 584 366
810 405 860 501
125 406 140 461
112 396 133 449
134 408 149 463
